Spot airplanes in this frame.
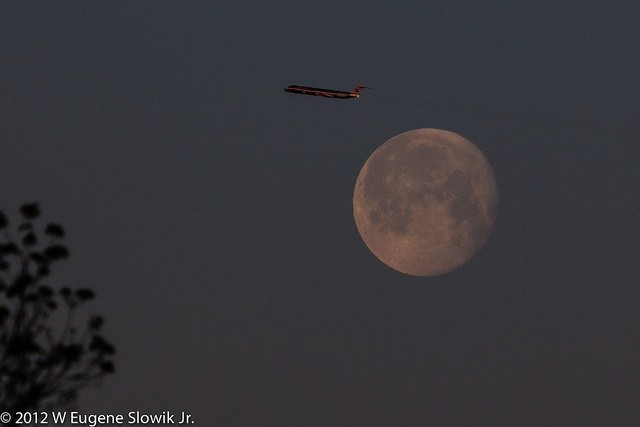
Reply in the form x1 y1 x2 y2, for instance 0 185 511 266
284 81 371 98
